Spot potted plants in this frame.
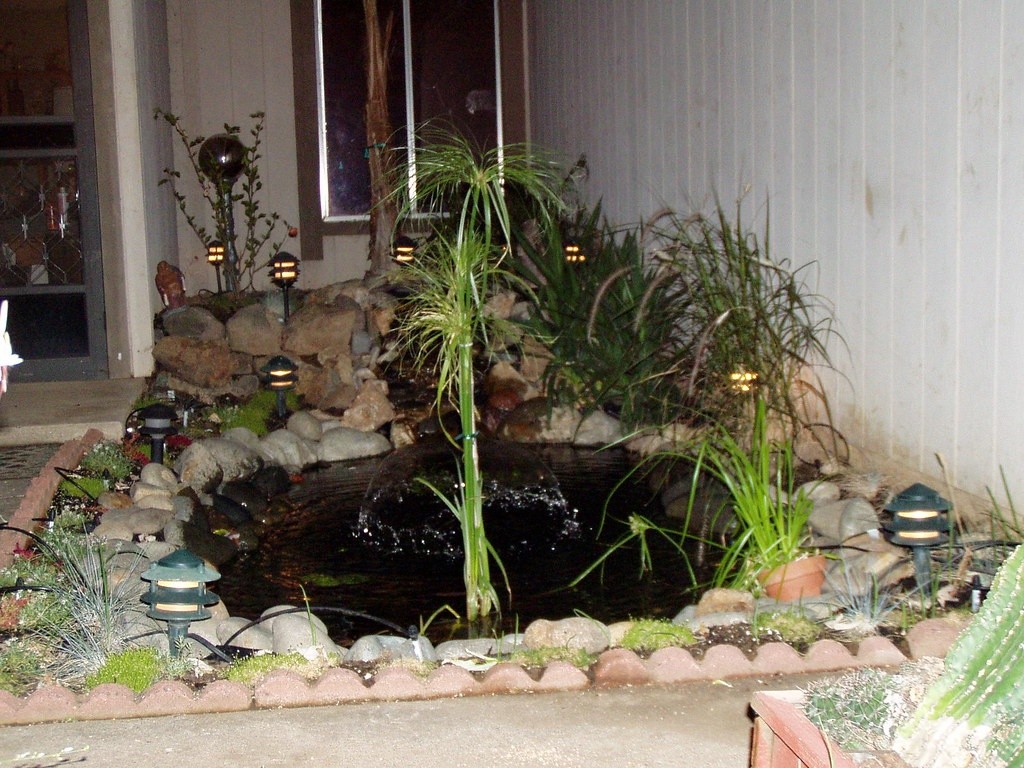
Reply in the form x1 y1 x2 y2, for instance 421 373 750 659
569 392 841 600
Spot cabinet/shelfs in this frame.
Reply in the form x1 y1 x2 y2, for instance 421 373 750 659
0 0 108 383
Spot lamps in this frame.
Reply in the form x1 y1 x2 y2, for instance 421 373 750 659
205 240 226 293
267 251 301 321
715 347 759 391
883 483 953 591
260 355 299 416
137 403 178 465
140 547 222 660
389 236 416 271
